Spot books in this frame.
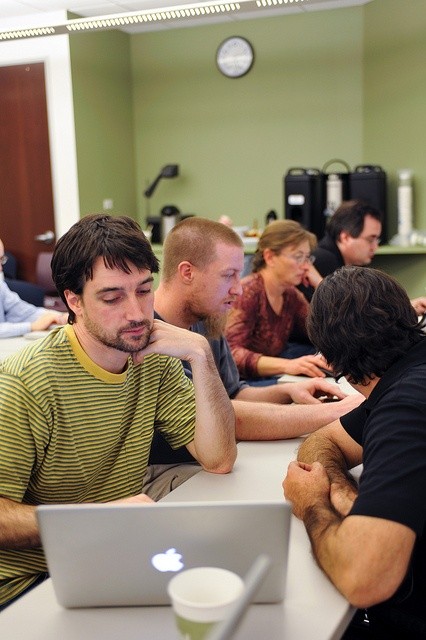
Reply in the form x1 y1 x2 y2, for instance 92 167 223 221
24 324 64 339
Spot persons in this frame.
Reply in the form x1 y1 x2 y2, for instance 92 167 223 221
295 200 426 314
0 239 70 339
0 213 238 611
152 216 367 442
223 219 334 378
283 267 424 640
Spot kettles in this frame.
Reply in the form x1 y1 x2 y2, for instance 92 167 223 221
160 204 180 245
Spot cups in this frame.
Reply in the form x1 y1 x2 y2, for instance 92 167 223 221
167 566 248 640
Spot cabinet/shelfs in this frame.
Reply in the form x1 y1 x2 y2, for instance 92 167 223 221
147 230 426 256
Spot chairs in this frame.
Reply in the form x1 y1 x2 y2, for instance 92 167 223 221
3 250 17 278
5 278 46 308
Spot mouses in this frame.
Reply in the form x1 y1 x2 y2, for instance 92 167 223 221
319 368 342 379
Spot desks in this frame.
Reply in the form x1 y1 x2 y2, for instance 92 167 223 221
0 373 364 640
0 304 71 362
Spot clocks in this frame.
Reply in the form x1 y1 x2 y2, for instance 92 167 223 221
216 34 254 78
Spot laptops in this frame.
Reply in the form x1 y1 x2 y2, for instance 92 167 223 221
33 500 293 609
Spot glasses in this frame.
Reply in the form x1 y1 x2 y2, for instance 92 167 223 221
362 238 381 245
269 251 316 264
